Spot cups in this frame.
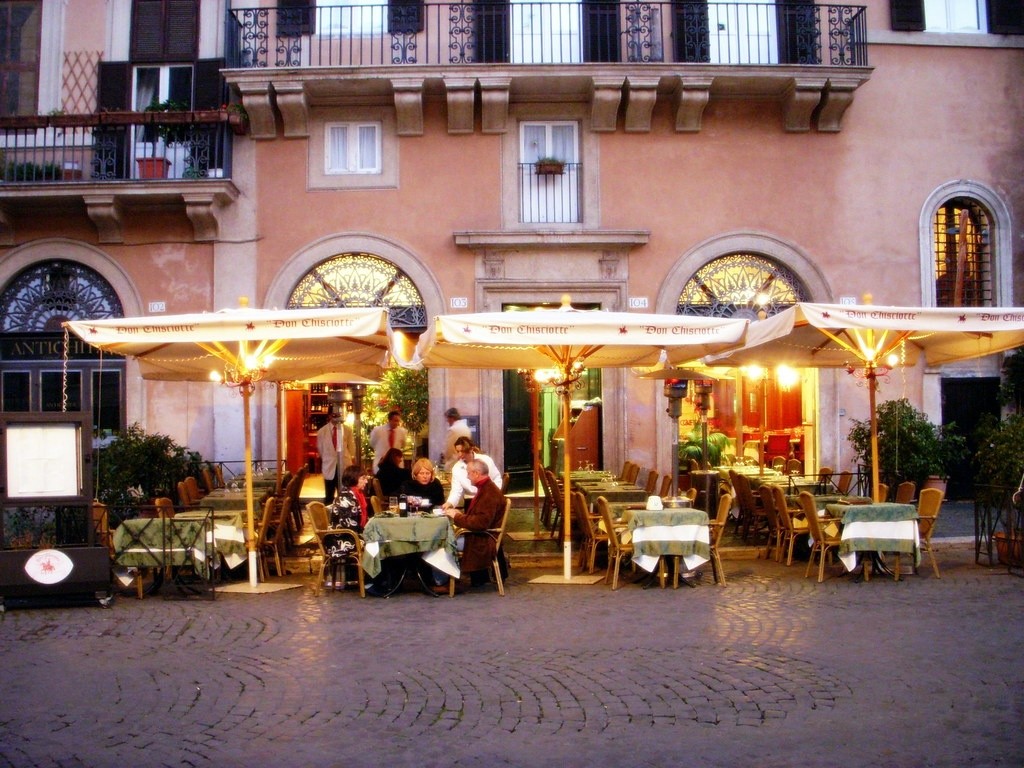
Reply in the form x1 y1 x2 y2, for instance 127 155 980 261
410 505 418 517
433 509 442 515
389 497 398 516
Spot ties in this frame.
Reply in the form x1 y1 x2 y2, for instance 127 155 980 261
388 429 395 448
332 426 337 451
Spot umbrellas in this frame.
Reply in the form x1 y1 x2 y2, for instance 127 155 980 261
701 290 1024 573
410 293 750 580
61 297 394 587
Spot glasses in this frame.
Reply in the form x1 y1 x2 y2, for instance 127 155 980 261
331 414 341 418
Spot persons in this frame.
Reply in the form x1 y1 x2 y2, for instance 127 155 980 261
369 411 407 475
404 458 445 507
326 464 383 597
371 448 406 514
441 436 503 513
443 406 472 474
431 458 505 593
317 406 355 506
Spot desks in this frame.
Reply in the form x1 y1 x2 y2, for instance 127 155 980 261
763 480 833 494
609 501 647 520
558 470 618 485
784 495 873 566
621 507 711 589
200 488 271 510
173 510 260 578
823 502 920 583
112 517 220 599
226 470 291 492
748 475 805 491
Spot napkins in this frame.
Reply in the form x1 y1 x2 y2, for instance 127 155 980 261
374 511 395 518
420 512 439 518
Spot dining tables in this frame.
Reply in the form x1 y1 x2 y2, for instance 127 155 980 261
575 480 646 514
358 510 461 598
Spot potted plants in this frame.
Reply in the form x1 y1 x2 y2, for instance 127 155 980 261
971 410 1024 566
676 420 731 493
847 398 966 500
109 421 183 517
534 155 565 174
136 98 191 179
0 506 110 610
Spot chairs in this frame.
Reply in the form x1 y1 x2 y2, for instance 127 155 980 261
794 434 805 475
764 434 794 468
93 456 944 601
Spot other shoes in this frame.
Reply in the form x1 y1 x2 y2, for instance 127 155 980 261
432 583 450 593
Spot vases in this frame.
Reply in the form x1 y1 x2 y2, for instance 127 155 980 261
207 169 223 179
60 169 82 180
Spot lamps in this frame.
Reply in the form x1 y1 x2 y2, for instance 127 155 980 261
533 361 585 386
245 353 258 384
251 352 273 382
208 371 242 387
875 354 899 384
841 359 865 379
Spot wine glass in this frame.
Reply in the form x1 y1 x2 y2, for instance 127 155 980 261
601 470 618 487
735 457 744 466
746 459 754 471
225 462 268 493
774 465 800 484
578 461 595 474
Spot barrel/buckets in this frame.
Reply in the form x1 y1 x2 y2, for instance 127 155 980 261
135 157 172 179
64 169 81 181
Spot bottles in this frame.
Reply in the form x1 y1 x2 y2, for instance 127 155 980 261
312 383 328 392
348 402 353 410
399 494 407 517
311 417 320 430
311 396 342 414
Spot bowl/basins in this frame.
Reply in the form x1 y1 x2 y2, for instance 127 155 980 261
421 504 434 514
